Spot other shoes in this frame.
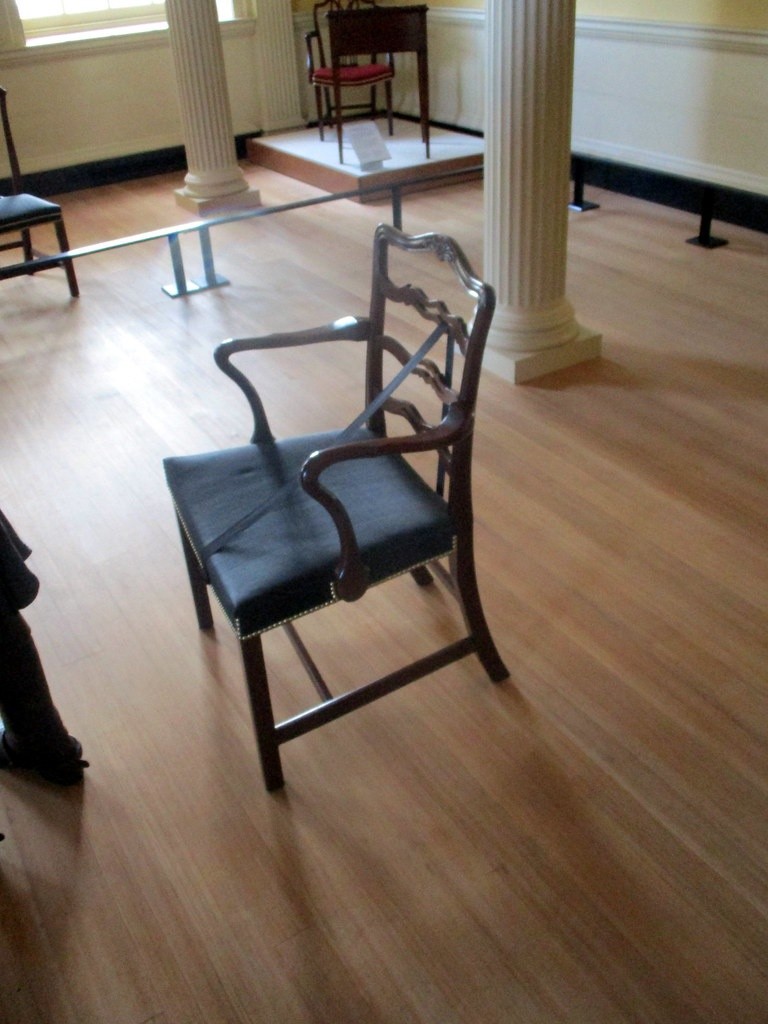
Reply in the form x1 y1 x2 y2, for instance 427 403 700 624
0 729 89 787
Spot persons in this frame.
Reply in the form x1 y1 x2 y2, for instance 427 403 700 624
0 507 90 843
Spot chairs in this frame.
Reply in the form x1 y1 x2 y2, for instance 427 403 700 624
0 87 79 297
304 0 395 142
163 224 508 793
